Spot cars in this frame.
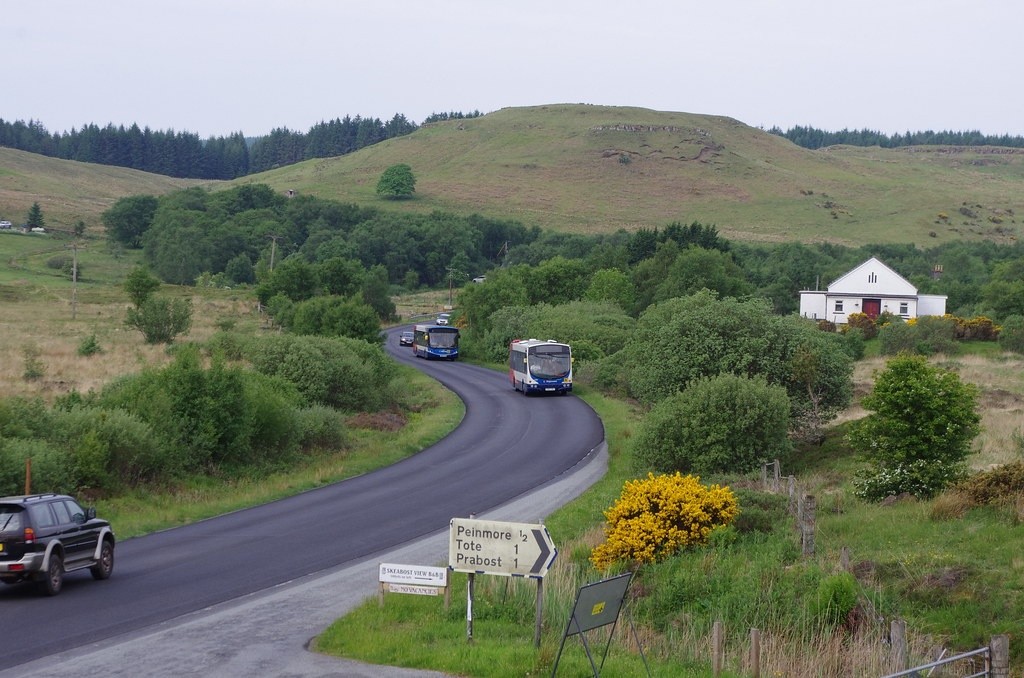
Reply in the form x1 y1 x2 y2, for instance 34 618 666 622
400 332 414 345
436 313 451 325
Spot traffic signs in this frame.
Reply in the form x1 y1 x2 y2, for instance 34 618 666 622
379 563 448 596
448 518 558 578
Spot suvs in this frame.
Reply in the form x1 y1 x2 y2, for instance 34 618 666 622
0 492 114 596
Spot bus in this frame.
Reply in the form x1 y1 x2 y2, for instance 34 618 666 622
412 324 459 361
509 339 574 395
